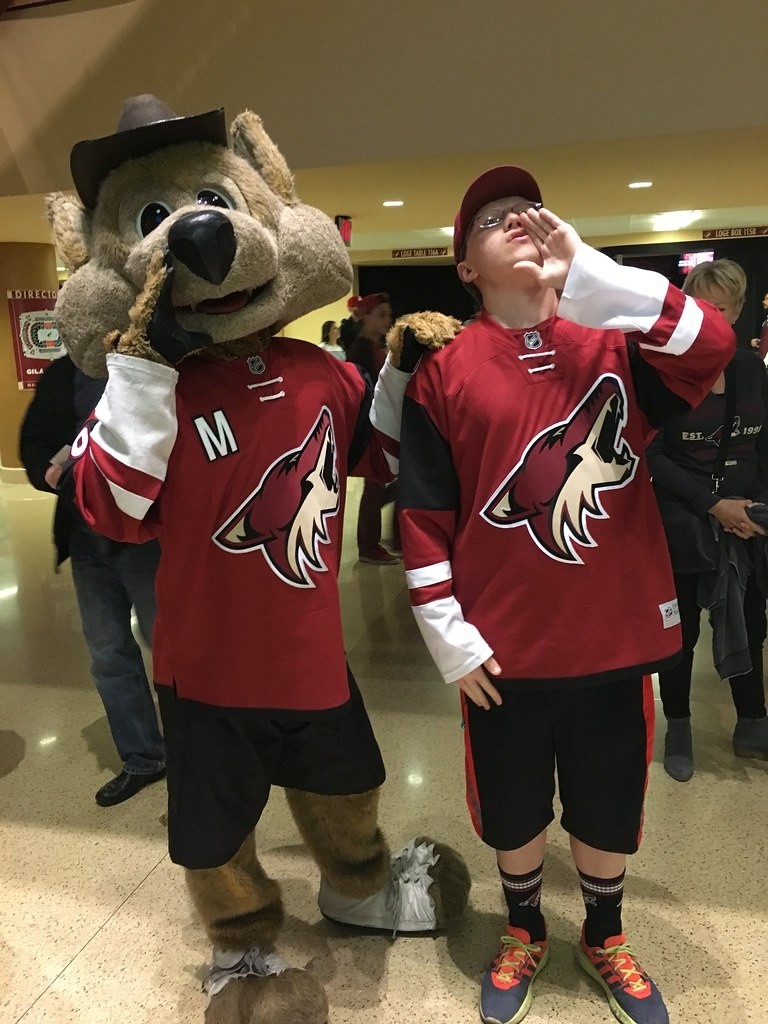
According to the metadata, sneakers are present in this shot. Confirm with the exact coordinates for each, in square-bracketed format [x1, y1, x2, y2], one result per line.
[575, 918, 669, 1024]
[479, 914, 550, 1024]
[358, 545, 400, 565]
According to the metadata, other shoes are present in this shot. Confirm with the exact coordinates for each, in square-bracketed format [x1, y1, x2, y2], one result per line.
[733, 716, 768, 761]
[664, 717, 694, 783]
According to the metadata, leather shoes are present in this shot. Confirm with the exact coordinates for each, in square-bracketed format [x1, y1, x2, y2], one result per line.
[95, 768, 166, 807]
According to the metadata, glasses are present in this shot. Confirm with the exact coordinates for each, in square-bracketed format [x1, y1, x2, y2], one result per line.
[462, 201, 541, 260]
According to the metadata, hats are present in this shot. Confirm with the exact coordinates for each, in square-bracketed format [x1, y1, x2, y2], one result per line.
[454, 166, 544, 265]
[348, 293, 390, 314]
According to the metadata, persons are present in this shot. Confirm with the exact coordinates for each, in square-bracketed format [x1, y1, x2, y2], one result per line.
[322, 292, 402, 567]
[648, 259, 768, 783]
[397, 165, 738, 1023]
[15, 356, 167, 807]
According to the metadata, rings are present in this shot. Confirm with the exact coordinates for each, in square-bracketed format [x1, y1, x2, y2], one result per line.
[740, 522, 750, 529]
[723, 527, 737, 532]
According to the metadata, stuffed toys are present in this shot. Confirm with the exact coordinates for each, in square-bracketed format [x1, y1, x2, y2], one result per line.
[46, 92, 470, 1024]
